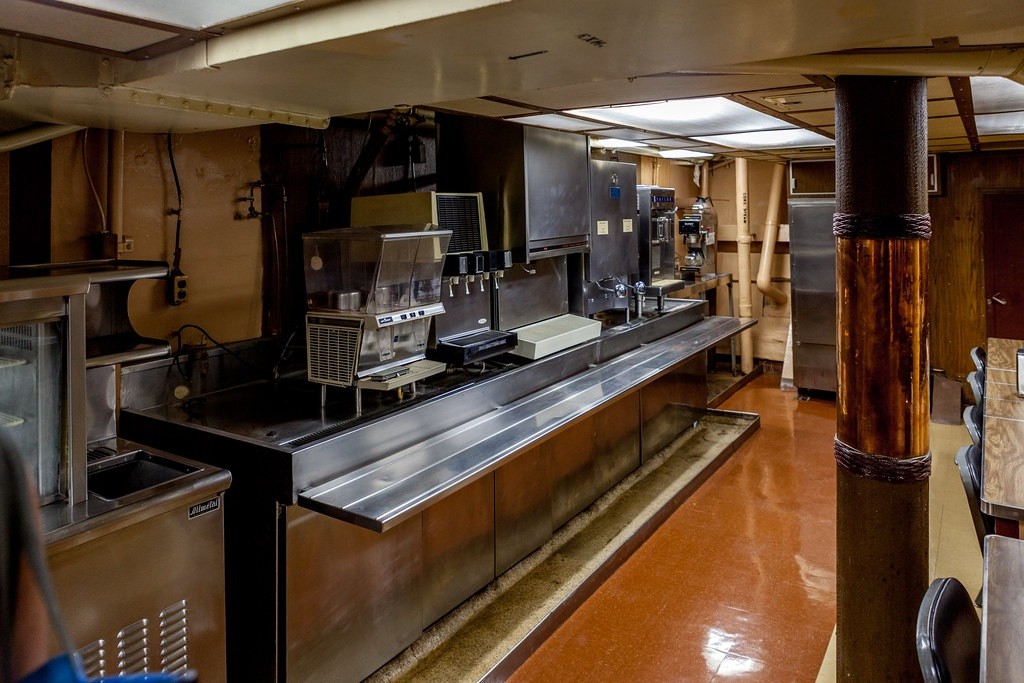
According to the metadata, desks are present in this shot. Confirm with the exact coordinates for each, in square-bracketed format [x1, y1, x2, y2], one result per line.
[979, 534, 1024, 683]
[981, 337, 1024, 523]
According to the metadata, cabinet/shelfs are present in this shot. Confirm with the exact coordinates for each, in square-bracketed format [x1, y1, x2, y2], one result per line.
[277, 350, 707, 683]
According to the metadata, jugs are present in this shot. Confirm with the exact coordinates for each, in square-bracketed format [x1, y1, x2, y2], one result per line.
[684, 247, 705, 266]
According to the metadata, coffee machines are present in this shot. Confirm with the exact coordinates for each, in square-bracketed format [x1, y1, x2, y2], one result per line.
[680, 197, 716, 280]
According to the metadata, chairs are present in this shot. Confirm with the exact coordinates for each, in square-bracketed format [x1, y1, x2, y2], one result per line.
[917, 345, 986, 683]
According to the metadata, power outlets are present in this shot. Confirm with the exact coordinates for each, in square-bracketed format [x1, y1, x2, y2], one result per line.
[167, 269, 188, 306]
